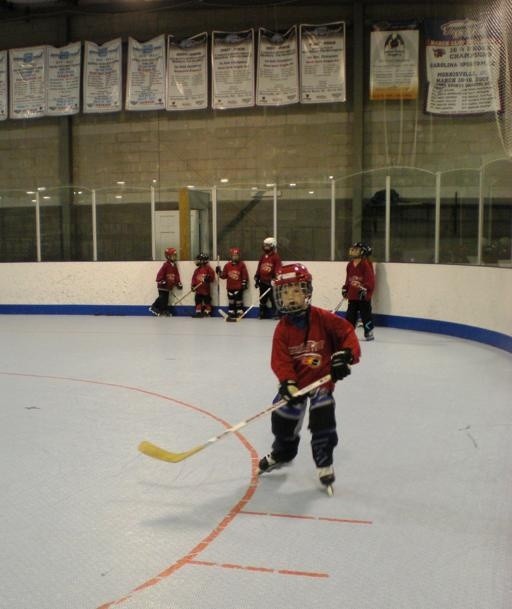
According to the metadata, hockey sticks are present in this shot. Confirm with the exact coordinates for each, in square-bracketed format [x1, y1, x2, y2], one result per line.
[218, 256, 229, 317]
[138, 372, 333, 463]
[226, 287, 271, 322]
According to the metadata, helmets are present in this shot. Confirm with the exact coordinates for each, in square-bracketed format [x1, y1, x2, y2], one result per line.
[230, 248, 240, 255]
[349, 241, 373, 259]
[193, 252, 209, 266]
[263, 237, 277, 248]
[165, 247, 176, 258]
[272, 263, 313, 314]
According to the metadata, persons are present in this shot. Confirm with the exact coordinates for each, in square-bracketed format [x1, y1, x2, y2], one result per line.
[259, 263, 362, 487]
[342, 242, 375, 341]
[148, 247, 182, 316]
[253, 237, 283, 319]
[190, 253, 215, 317]
[216, 246, 249, 322]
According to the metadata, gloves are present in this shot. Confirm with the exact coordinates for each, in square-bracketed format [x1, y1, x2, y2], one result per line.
[160, 280, 167, 287]
[342, 286, 346, 296]
[255, 280, 261, 288]
[329, 346, 352, 383]
[204, 276, 211, 282]
[358, 290, 366, 300]
[175, 282, 183, 289]
[279, 379, 307, 405]
[239, 281, 248, 290]
[191, 286, 196, 292]
[259, 454, 278, 470]
[215, 266, 222, 274]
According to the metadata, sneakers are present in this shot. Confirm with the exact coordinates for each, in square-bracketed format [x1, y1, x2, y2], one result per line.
[364, 331, 374, 341]
[316, 463, 335, 485]
[149, 304, 211, 316]
[227, 303, 275, 317]
